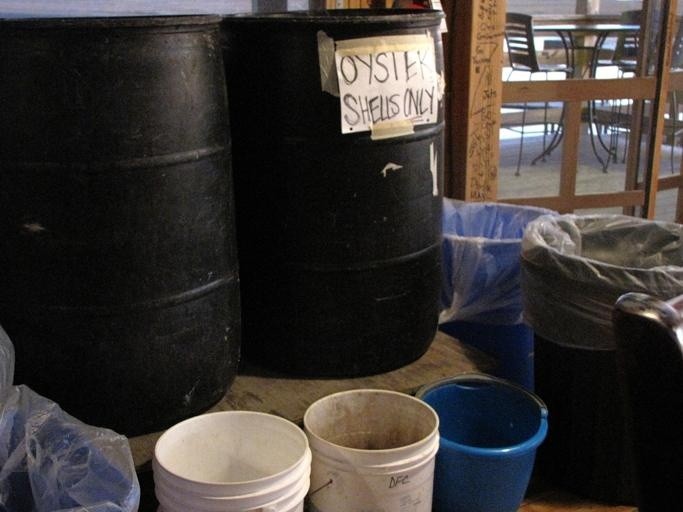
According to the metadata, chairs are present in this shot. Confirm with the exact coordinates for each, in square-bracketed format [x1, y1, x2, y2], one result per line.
[501, 12, 683, 177]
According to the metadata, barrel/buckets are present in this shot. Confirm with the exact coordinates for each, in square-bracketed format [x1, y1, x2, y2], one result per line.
[152, 411, 312, 511]
[0, 17, 244, 435]
[303, 388, 440, 512]
[220, 4, 446, 378]
[414, 369, 548, 512]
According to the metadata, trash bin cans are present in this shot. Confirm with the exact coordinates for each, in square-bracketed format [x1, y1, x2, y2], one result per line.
[610, 290, 683, 512]
[520, 213, 682, 508]
[0, 408, 136, 512]
[442, 196, 560, 394]
